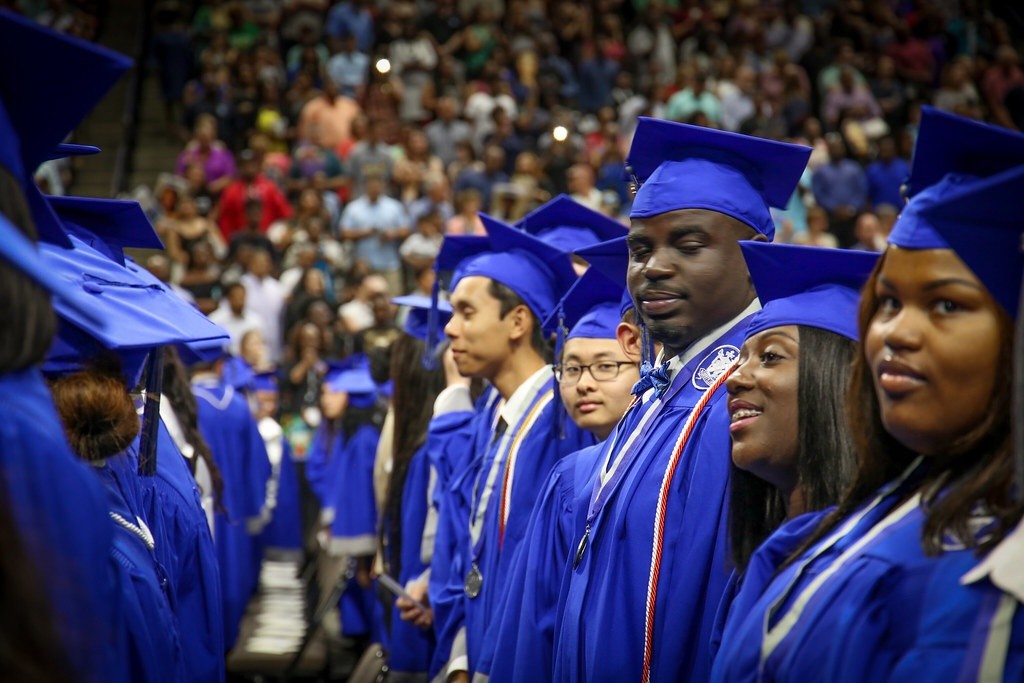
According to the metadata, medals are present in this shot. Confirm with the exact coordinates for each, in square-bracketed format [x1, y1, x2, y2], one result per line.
[465, 564, 483, 599]
[573, 533, 589, 570]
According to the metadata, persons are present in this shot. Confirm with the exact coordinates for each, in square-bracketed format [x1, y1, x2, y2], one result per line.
[0, 0, 1024, 683]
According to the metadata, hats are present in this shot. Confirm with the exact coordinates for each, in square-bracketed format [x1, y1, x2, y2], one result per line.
[541, 265, 624, 368]
[1, 12, 259, 391]
[463, 212, 577, 340]
[511, 192, 629, 256]
[738, 240, 884, 343]
[321, 356, 381, 408]
[572, 234, 655, 369]
[422, 235, 489, 369]
[918, 168, 1023, 313]
[627, 116, 814, 240]
[390, 296, 453, 343]
[886, 103, 1024, 251]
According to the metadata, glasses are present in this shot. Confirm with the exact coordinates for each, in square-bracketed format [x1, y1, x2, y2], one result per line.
[552, 359, 640, 382]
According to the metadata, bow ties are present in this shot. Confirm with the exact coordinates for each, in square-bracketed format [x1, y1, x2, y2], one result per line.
[630, 360, 672, 399]
